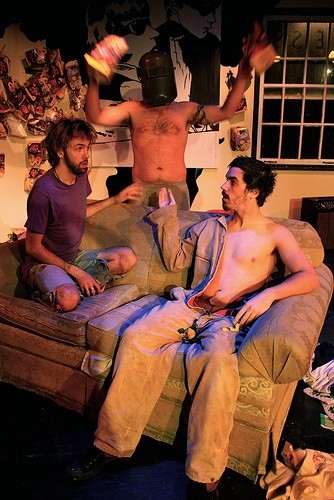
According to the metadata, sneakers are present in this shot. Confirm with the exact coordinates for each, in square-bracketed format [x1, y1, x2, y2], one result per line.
[66, 440, 123, 480]
[187, 477, 221, 500]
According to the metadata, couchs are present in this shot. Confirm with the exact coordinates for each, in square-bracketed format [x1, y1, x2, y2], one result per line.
[0, 199, 334, 486]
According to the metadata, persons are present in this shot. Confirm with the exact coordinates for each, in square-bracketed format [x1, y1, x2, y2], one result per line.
[64, 155, 320, 500]
[20, 117, 137, 313]
[83, 29, 265, 212]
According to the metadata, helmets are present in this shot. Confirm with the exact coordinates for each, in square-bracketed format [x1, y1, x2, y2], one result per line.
[136, 51, 178, 106]
